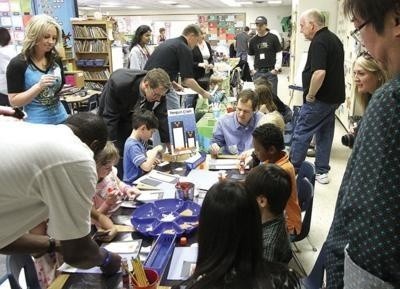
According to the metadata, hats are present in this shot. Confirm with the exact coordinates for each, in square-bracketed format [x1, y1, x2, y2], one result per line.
[256, 16, 267, 24]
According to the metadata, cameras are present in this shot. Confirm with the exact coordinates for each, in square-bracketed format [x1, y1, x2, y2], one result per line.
[342, 133, 354, 147]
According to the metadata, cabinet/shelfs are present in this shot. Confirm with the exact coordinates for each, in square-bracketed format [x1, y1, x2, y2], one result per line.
[70, 20, 114, 85]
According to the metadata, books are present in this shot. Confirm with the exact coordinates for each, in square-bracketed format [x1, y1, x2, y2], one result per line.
[72, 24, 108, 88]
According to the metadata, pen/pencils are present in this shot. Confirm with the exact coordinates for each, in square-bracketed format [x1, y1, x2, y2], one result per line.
[121, 255, 151, 287]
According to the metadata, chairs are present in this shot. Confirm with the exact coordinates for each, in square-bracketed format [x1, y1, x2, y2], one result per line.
[280, 160, 319, 252]
[73, 93, 99, 113]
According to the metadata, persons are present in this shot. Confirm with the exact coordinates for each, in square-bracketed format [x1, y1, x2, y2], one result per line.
[91, 140, 142, 243]
[6, 13, 72, 125]
[0, 112, 122, 276]
[287, 6, 347, 186]
[322, 1, 400, 289]
[347, 50, 386, 140]
[0, 104, 26, 120]
[237, 123, 302, 242]
[99, 11, 294, 181]
[243, 162, 295, 266]
[0, 27, 19, 107]
[170, 180, 315, 289]
[27, 211, 118, 289]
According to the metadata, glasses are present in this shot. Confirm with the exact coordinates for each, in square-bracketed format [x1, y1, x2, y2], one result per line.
[350, 18, 370, 42]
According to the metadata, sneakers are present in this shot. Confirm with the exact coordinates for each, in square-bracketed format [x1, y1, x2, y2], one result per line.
[315, 173, 329, 183]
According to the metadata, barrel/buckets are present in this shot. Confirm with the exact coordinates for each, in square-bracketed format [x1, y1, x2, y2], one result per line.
[175, 181, 196, 200]
[129, 266, 160, 289]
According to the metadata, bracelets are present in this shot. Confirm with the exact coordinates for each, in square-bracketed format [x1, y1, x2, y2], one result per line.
[100, 250, 113, 268]
[49, 236, 58, 253]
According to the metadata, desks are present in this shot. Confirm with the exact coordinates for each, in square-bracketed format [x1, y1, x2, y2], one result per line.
[195, 112, 217, 139]
[49, 154, 234, 289]
[64, 90, 103, 113]
